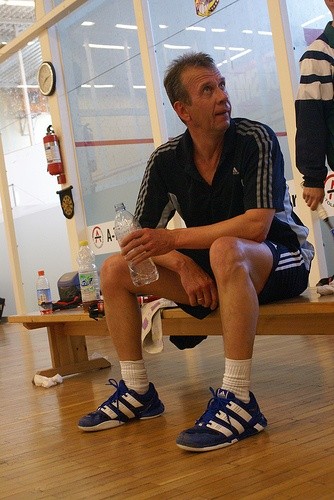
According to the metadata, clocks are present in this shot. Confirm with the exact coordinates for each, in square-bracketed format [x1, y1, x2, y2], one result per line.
[38, 62, 55, 96]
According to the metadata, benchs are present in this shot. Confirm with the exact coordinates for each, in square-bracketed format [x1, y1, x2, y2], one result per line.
[9, 287, 334, 386]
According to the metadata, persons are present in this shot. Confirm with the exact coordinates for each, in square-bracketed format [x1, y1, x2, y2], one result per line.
[294, 0, 334, 210]
[76, 52, 315, 453]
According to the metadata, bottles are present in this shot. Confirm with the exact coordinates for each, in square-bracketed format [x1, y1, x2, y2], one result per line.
[77, 240, 100, 302]
[113, 203, 160, 286]
[36, 270, 52, 310]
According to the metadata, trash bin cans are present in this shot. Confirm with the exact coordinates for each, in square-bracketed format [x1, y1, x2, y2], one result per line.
[56, 271, 82, 304]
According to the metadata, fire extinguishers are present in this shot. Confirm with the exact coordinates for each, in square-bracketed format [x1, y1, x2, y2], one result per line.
[42, 125, 64, 175]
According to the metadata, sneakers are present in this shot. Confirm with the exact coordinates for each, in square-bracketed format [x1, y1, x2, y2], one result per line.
[78, 378, 165, 432]
[175, 385, 268, 452]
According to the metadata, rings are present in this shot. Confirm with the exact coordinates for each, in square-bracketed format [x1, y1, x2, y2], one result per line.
[197, 297, 204, 301]
